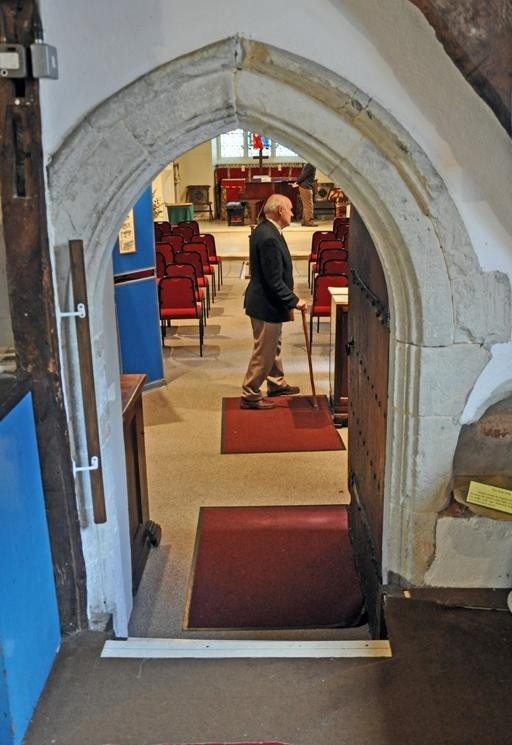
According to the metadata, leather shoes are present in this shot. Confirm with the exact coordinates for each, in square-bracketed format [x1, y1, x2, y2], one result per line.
[267, 384, 300, 397]
[239, 395, 276, 409]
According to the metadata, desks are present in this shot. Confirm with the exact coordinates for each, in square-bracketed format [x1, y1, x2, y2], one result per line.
[244, 177, 300, 226]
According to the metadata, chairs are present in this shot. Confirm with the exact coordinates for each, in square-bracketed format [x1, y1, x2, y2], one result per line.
[312, 182, 336, 219]
[307, 214, 349, 351]
[154, 220, 223, 360]
[184, 185, 214, 221]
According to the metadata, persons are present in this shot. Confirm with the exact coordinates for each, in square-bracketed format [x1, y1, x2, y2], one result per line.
[293, 162, 319, 227]
[237, 193, 309, 409]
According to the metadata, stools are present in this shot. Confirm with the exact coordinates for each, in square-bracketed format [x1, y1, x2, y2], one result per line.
[226, 200, 244, 226]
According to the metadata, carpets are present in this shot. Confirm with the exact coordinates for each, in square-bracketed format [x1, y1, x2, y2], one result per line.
[221, 393, 345, 454]
[180, 502, 367, 633]
